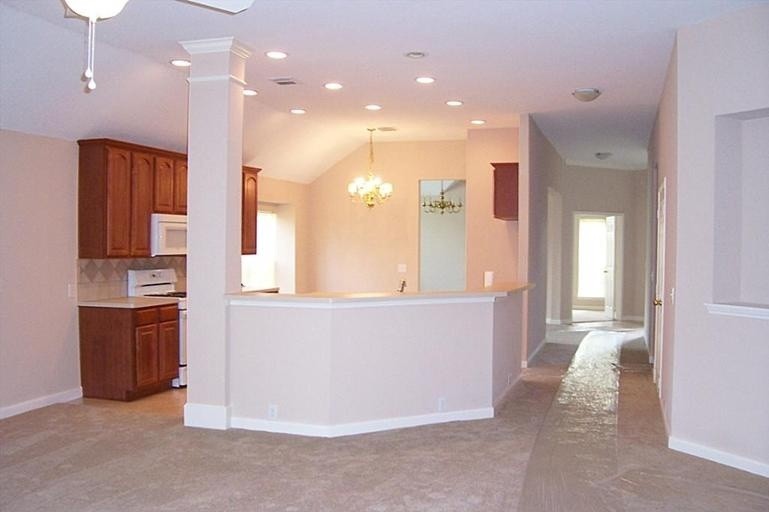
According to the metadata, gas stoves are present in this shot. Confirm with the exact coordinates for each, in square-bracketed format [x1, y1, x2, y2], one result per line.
[127, 290, 188, 309]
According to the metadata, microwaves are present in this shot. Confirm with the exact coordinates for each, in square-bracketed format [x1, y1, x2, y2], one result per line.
[151, 214, 187, 258]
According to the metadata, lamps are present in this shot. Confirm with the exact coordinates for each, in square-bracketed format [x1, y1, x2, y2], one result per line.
[63, 0, 130, 20]
[348, 129, 394, 209]
[422, 180, 463, 215]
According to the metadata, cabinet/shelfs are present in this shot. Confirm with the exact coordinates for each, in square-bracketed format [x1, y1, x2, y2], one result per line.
[78, 139, 258, 260]
[79, 305, 179, 402]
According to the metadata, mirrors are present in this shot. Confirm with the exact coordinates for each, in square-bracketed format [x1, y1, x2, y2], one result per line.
[420, 180, 466, 293]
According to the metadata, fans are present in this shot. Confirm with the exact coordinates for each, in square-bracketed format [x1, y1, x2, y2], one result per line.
[58, 0, 253, 14]
